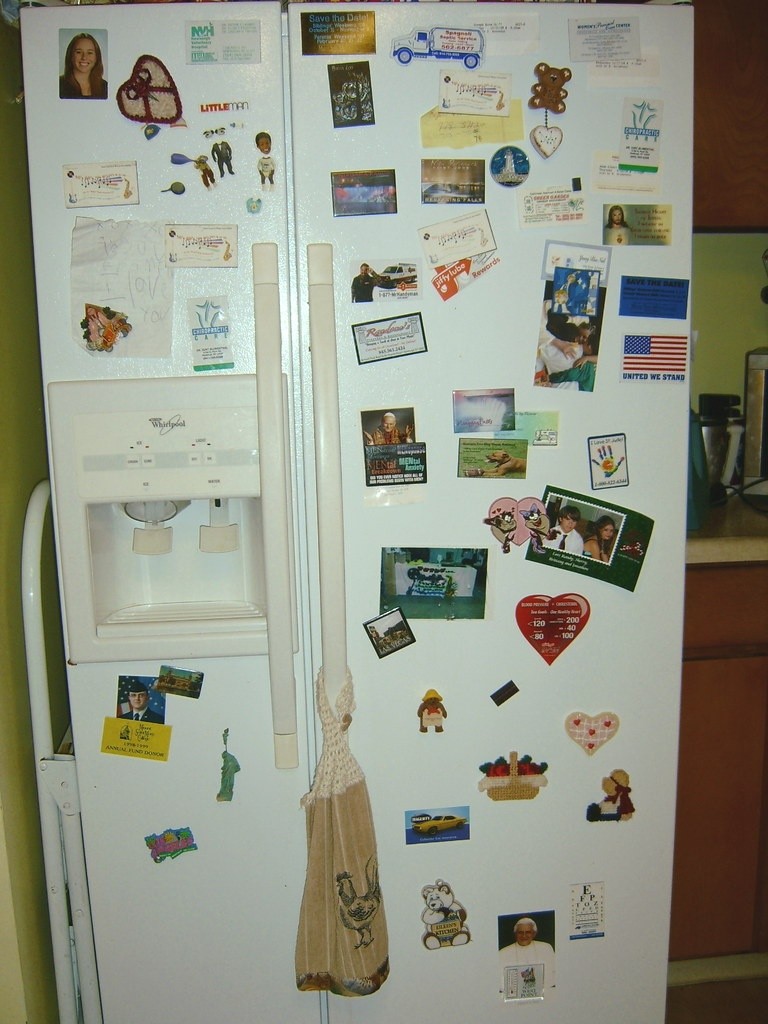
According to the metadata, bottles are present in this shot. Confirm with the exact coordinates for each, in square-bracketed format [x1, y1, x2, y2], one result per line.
[687, 395, 709, 531]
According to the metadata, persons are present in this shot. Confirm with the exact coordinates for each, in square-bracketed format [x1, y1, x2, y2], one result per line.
[117, 680, 165, 724]
[59, 28, 108, 100]
[540, 506, 585, 556]
[362, 412, 414, 446]
[255, 133, 271, 155]
[603, 205, 634, 245]
[498, 917, 555, 984]
[538, 300, 599, 391]
[351, 263, 391, 303]
[583, 514, 615, 562]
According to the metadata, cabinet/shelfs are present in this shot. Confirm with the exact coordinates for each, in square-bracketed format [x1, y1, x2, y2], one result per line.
[666, 561, 768, 987]
[692, 0, 768, 231]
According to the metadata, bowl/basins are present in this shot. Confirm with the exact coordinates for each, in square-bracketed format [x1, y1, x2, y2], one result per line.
[462, 468, 485, 477]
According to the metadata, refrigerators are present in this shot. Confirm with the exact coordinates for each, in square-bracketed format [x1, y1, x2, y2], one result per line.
[19, 1, 697, 1024]
